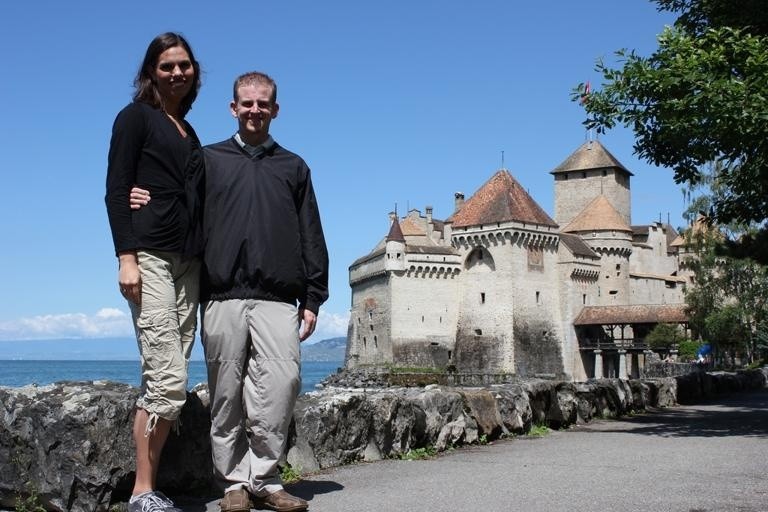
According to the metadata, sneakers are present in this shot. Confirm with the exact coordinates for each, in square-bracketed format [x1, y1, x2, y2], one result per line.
[253, 491, 308, 512]
[128, 490, 183, 512]
[222, 488, 252, 512]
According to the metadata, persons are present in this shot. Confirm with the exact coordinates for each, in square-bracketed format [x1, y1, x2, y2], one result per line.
[129, 73, 330, 512]
[103, 33, 206, 511]
